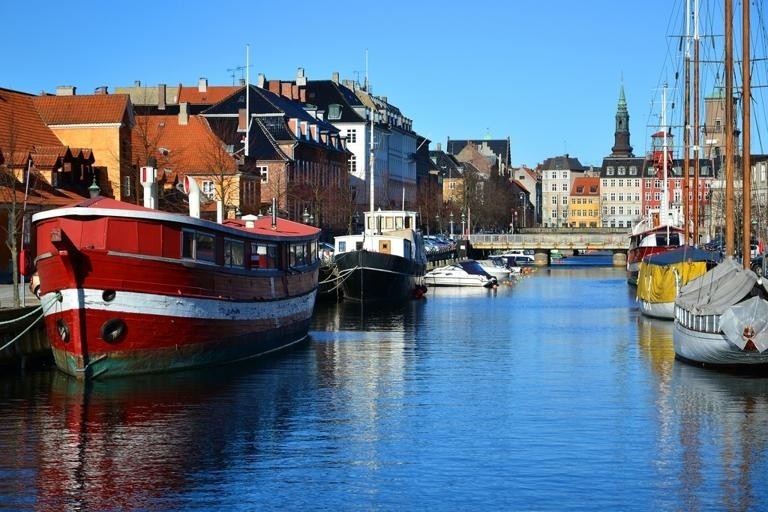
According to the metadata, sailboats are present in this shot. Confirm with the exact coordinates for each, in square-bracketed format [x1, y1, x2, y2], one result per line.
[625, 80, 703, 286]
[672, 1, 768, 366]
[636, 2, 727, 320]
[704, 7, 768, 297]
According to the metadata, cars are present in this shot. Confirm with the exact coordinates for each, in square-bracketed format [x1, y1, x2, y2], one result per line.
[423, 233, 456, 252]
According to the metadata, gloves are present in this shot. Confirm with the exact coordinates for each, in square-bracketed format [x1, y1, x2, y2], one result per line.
[333, 209, 426, 303]
[424, 250, 535, 286]
[32, 165, 322, 378]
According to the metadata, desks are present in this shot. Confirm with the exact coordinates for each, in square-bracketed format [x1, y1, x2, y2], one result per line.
[511, 208, 517, 234]
[461, 212, 467, 234]
[449, 212, 455, 234]
[520, 192, 526, 227]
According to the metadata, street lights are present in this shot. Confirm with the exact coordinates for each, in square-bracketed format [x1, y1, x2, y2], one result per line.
[511, 208, 517, 234]
[449, 212, 455, 234]
[461, 212, 467, 234]
[520, 192, 526, 227]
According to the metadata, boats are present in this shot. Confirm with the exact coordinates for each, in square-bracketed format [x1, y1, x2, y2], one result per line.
[32, 165, 322, 378]
[424, 250, 535, 286]
[333, 209, 426, 303]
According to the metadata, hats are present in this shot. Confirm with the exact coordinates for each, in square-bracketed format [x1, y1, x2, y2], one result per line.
[423, 233, 456, 252]
